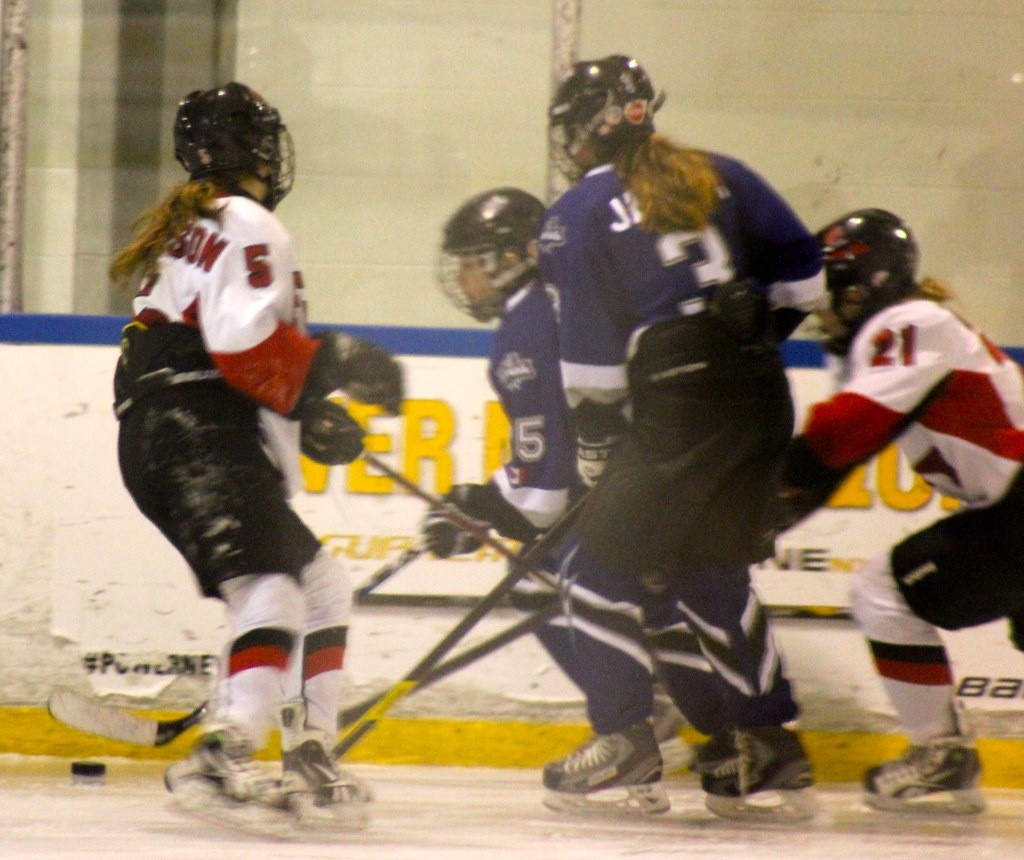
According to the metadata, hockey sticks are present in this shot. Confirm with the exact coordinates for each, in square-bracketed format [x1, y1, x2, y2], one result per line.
[336, 601, 565, 730]
[327, 485, 601, 766]
[357, 450, 562, 593]
[44, 530, 440, 750]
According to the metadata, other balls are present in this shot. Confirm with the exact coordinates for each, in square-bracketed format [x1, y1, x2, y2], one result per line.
[70, 760, 107, 778]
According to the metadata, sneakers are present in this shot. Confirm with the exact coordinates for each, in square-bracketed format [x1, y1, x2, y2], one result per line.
[541, 715, 671, 816]
[165, 723, 308, 841]
[696, 721, 818, 826]
[864, 696, 985, 823]
[646, 686, 692, 778]
[280, 705, 372, 820]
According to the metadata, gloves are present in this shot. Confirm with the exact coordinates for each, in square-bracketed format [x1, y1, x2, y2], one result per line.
[312, 329, 399, 415]
[425, 484, 491, 557]
[773, 436, 852, 531]
[300, 400, 366, 465]
[570, 404, 632, 489]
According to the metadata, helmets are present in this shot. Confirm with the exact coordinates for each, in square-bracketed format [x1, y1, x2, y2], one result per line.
[817, 209, 918, 325]
[174, 83, 295, 210]
[437, 188, 549, 322]
[547, 55, 656, 185]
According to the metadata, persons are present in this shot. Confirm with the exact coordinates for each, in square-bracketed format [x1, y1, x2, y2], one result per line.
[425, 54, 1023, 824]
[108, 81, 401, 836]
